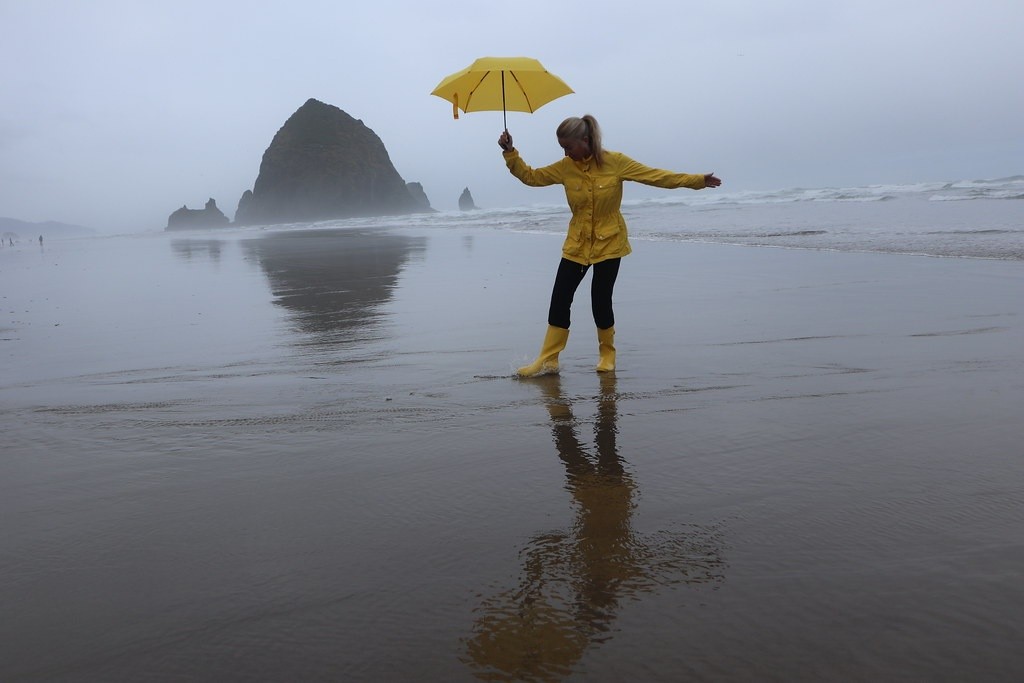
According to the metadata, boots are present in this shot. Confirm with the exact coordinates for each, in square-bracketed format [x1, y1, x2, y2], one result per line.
[596, 324, 616, 372]
[516, 324, 570, 376]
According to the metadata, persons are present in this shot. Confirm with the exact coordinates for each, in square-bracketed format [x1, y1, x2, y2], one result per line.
[498, 114, 723, 379]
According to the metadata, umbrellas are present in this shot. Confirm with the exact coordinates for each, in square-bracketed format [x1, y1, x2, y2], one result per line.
[430, 55, 575, 144]
